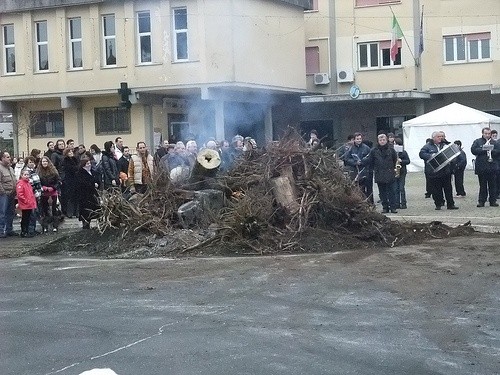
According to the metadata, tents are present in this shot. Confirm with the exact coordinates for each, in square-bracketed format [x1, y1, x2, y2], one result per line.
[402, 101, 500, 173]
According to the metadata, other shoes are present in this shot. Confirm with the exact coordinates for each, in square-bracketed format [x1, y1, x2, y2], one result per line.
[44, 228, 47, 231]
[5, 230, 19, 236]
[425, 194, 430, 198]
[456, 192, 466, 196]
[53, 228, 57, 232]
[400, 205, 406, 209]
[447, 205, 459, 209]
[391, 209, 397, 213]
[477, 201, 484, 207]
[382, 209, 389, 213]
[0, 233, 6, 237]
[435, 206, 441, 210]
[68, 215, 72, 218]
[490, 201, 499, 206]
[76, 215, 79, 217]
[21, 231, 40, 238]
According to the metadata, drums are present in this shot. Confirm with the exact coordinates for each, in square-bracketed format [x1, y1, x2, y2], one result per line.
[426, 142, 462, 173]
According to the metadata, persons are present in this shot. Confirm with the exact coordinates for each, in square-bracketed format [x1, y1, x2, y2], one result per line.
[101, 140, 122, 193]
[471, 127, 499, 207]
[340, 132, 373, 204]
[36, 156, 63, 234]
[0, 135, 259, 237]
[361, 130, 398, 213]
[15, 168, 38, 237]
[127, 141, 156, 196]
[0, 150, 20, 237]
[75, 159, 103, 230]
[419, 131, 459, 210]
[305, 130, 499, 208]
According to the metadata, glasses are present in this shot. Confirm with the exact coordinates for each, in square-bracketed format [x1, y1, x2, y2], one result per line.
[138, 147, 145, 149]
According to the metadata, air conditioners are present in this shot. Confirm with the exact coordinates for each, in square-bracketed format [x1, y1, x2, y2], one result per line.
[313, 73, 330, 85]
[337, 68, 354, 83]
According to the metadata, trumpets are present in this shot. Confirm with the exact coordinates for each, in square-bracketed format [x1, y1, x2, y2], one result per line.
[395, 152, 402, 178]
[485, 139, 493, 163]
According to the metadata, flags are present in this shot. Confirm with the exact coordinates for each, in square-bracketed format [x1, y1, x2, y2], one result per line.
[391, 16, 424, 63]
